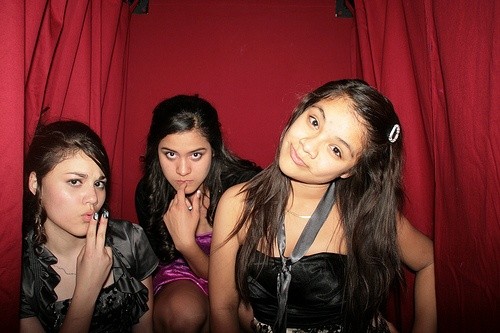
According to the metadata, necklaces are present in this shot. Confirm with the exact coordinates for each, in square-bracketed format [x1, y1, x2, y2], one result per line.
[53, 264, 77, 277]
[286, 209, 311, 218]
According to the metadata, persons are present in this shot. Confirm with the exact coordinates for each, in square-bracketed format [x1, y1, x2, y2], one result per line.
[209, 78, 437, 333]
[19, 120, 158, 333]
[135, 94, 264, 333]
